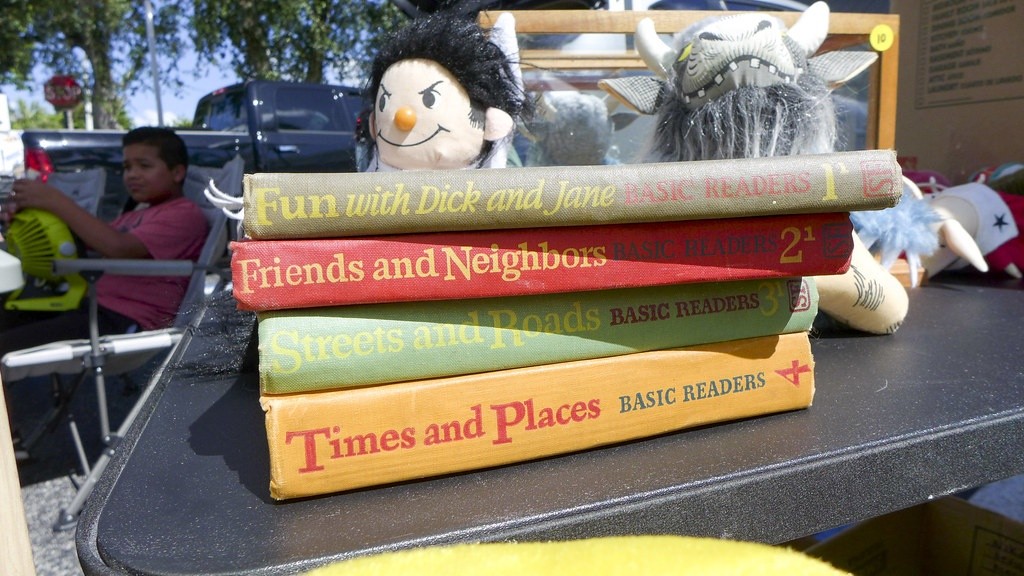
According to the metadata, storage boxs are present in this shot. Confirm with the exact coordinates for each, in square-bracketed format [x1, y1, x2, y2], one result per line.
[888, 0, 1024, 186]
[777, 494, 1024, 576]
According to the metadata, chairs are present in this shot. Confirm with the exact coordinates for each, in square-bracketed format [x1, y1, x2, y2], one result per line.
[0, 151, 244, 490]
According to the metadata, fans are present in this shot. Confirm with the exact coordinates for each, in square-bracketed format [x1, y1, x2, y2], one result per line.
[4, 207, 88, 311]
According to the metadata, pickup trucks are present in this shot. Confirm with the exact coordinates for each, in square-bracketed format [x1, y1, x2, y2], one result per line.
[21, 77, 375, 232]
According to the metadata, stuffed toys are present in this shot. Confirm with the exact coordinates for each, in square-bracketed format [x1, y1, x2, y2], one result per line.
[354, 0, 1024, 335]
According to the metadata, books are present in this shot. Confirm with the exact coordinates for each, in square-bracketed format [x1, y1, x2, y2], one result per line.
[228, 147, 905, 502]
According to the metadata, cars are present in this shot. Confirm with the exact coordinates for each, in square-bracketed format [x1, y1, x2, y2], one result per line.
[505, 0, 869, 168]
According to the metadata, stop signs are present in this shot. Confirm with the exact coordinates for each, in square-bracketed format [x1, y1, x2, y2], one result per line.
[44, 74, 84, 111]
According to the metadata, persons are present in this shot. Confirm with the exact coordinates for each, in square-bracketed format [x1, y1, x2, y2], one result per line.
[0, 126, 210, 461]
